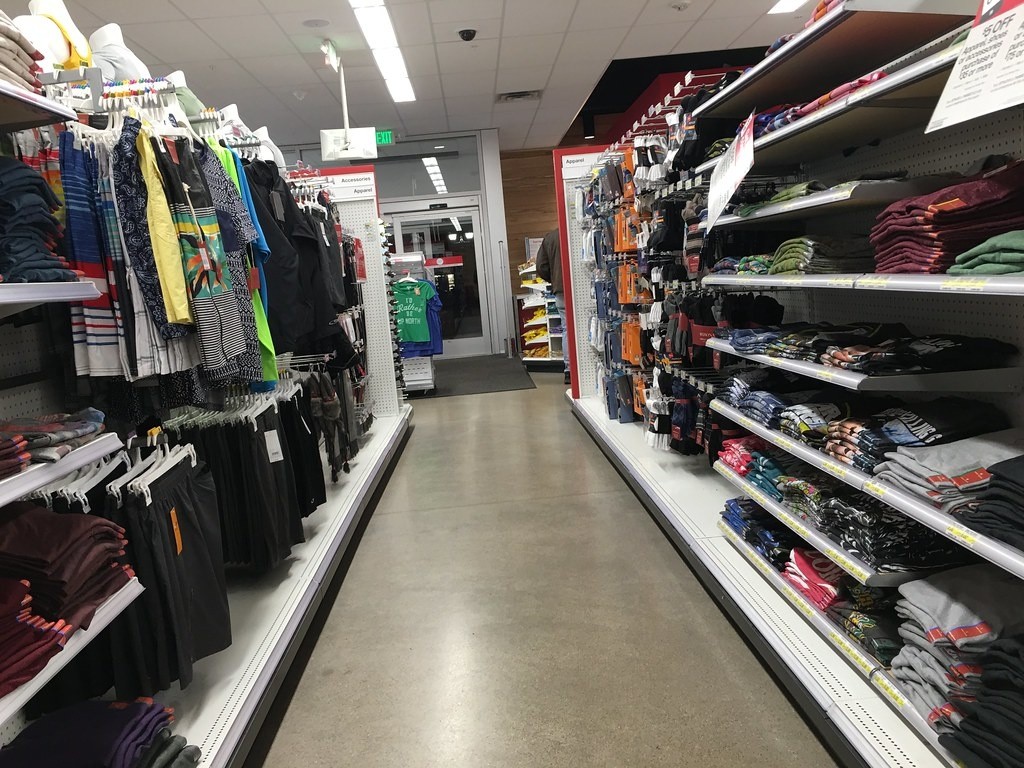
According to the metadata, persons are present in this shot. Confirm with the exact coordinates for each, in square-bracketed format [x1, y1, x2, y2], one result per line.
[536, 228, 571, 385]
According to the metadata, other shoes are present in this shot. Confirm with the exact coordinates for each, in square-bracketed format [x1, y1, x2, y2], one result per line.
[565, 371, 571, 384]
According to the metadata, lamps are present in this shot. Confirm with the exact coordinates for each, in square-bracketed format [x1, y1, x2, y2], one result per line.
[583, 111, 596, 140]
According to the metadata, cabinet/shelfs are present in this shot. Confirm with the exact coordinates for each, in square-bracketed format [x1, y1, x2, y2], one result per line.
[516, 260, 564, 374]
[704, 2, 1024, 768]
[1, 80, 149, 724]
